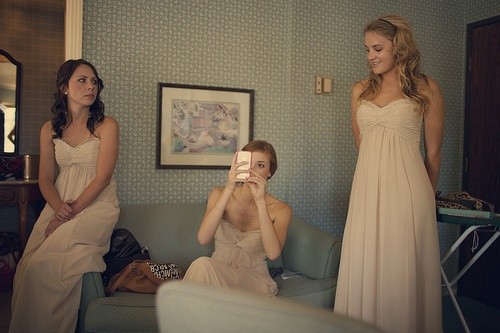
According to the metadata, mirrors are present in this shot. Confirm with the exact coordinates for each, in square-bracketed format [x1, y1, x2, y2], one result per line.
[0, 49, 21, 156]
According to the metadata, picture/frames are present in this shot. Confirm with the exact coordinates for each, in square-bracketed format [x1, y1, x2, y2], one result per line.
[156, 82, 255, 170]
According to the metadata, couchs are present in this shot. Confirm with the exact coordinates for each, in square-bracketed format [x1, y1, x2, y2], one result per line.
[79, 203, 342, 333]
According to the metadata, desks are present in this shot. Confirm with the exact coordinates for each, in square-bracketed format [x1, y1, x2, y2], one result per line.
[436, 213, 500, 333]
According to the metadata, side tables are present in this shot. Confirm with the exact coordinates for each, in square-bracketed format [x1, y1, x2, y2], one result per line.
[0, 180, 41, 258]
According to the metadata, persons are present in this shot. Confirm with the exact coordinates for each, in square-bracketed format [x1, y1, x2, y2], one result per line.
[8, 128, 14, 144]
[334, 15, 444, 333]
[8, 59, 120, 333]
[182, 141, 292, 297]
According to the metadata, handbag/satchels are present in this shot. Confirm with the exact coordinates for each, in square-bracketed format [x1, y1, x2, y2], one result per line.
[105, 260, 181, 296]
[100, 229, 149, 284]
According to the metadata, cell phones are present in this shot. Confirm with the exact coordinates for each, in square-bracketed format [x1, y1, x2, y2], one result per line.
[236, 151, 252, 179]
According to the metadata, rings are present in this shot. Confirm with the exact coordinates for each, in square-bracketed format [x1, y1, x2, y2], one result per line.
[59, 212, 62, 215]
